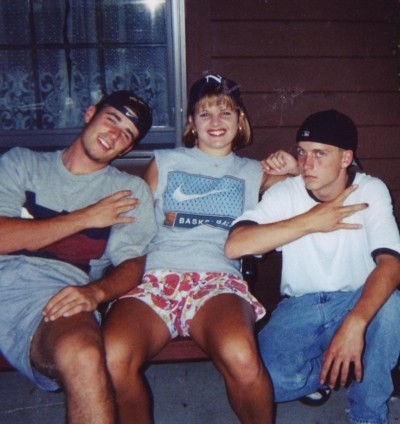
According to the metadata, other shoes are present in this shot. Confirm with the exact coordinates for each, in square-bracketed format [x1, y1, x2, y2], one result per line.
[299, 388, 333, 406]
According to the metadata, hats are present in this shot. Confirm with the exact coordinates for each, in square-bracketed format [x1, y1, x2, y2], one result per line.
[297, 111, 366, 173]
[103, 90, 153, 137]
[189, 75, 240, 103]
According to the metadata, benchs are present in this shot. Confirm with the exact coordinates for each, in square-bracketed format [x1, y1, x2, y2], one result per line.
[0, 249, 289, 370]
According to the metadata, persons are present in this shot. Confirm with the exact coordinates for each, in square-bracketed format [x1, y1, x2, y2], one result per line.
[100, 74, 300, 424]
[0, 86, 157, 423]
[225, 110, 400, 424]
